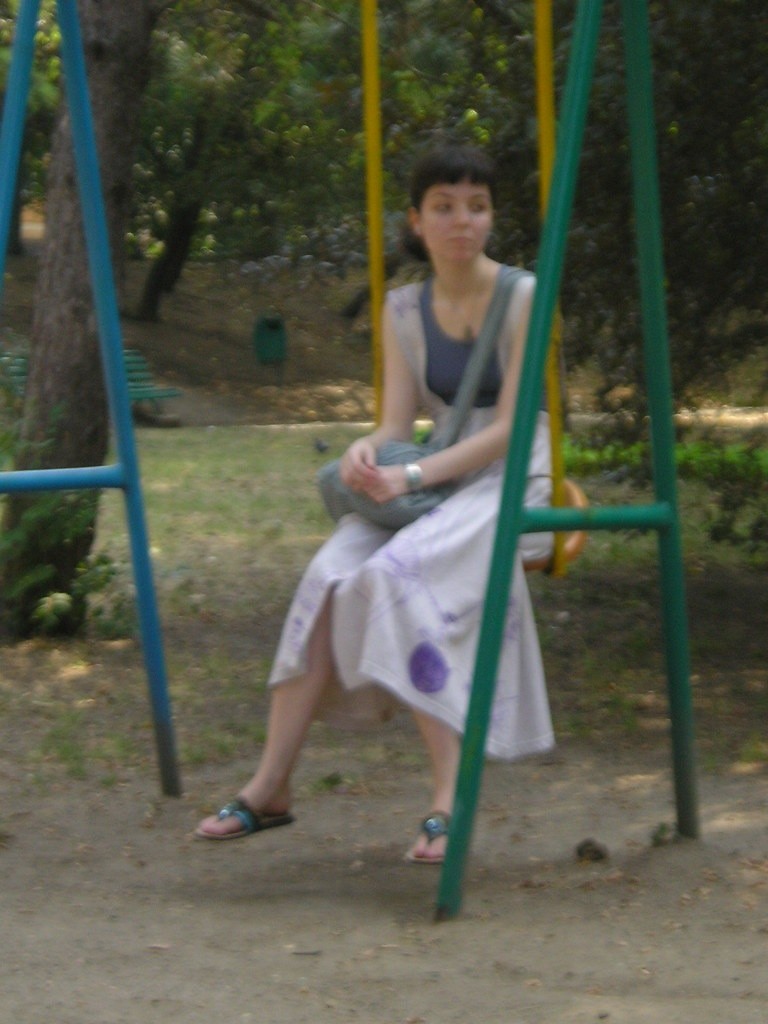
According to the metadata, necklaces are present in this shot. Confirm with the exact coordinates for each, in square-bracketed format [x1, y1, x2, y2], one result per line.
[433, 278, 485, 344]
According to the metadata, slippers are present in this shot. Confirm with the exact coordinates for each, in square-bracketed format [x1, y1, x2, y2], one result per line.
[193, 796, 296, 840]
[404, 809, 450, 862]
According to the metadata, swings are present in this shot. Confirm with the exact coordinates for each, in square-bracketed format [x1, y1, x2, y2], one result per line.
[362, 1, 590, 577]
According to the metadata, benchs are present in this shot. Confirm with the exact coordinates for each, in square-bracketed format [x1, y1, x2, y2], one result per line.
[0, 347, 182, 415]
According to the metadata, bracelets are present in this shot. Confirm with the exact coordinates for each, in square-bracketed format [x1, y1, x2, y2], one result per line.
[406, 463, 424, 491]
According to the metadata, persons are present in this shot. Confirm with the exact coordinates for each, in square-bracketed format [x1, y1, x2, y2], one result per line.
[196, 149, 554, 863]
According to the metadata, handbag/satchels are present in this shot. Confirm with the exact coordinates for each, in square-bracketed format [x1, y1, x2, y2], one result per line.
[315, 440, 454, 531]
